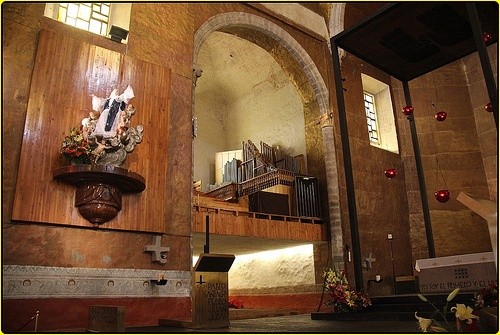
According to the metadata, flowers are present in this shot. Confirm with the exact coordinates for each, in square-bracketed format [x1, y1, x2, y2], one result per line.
[413, 281, 498, 334]
[322, 264, 372, 312]
[56, 128, 95, 166]
